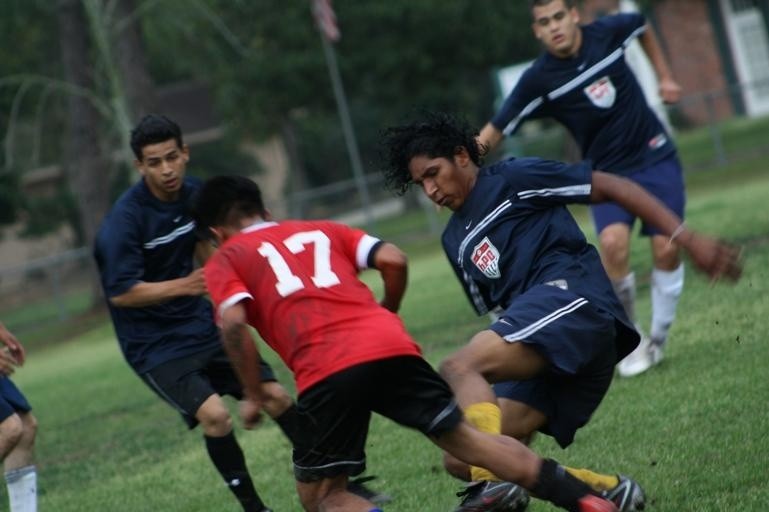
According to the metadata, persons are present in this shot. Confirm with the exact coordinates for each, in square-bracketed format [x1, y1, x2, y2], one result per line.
[0, 318, 40, 512]
[469, 1, 687, 381]
[374, 111, 744, 512]
[197, 171, 621, 512]
[93, 112, 393, 511]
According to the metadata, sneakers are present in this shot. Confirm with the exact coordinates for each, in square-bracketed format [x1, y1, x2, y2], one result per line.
[453, 477, 531, 512]
[577, 472, 646, 512]
[618, 342, 665, 377]
